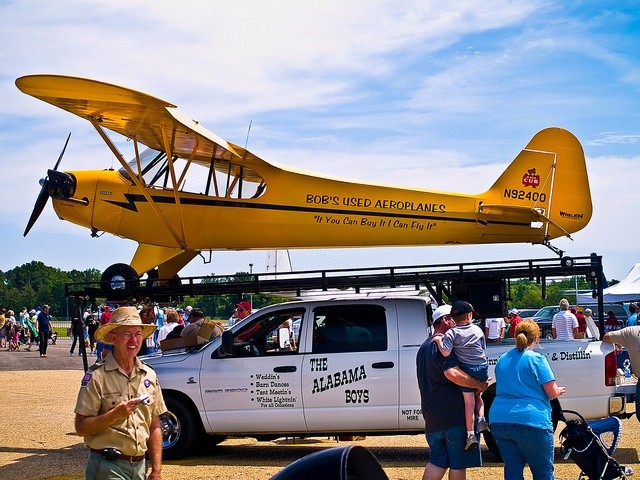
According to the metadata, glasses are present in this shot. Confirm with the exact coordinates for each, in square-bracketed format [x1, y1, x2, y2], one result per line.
[237, 308, 245, 312]
[115, 331, 145, 340]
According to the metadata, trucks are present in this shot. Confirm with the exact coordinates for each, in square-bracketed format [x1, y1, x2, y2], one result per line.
[523, 304, 628, 340]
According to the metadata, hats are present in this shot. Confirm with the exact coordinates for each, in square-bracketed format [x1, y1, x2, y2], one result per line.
[560, 298, 569, 310]
[43, 304, 51, 308]
[168, 310, 180, 322]
[510, 309, 518, 314]
[94, 306, 158, 345]
[29, 309, 36, 314]
[177, 309, 185, 313]
[452, 301, 480, 316]
[187, 308, 203, 322]
[432, 305, 452, 323]
[231, 301, 251, 314]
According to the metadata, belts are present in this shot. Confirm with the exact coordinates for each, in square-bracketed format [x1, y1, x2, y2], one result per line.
[89, 447, 146, 464]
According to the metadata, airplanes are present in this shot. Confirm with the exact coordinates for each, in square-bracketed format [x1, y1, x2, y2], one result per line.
[15, 75, 593, 299]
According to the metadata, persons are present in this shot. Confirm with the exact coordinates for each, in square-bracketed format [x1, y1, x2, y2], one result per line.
[181, 308, 222, 338]
[99, 306, 112, 325]
[433, 301, 491, 451]
[20, 306, 30, 329]
[504, 317, 511, 339]
[229, 309, 238, 327]
[603, 324, 640, 422]
[236, 301, 262, 342]
[36, 305, 52, 358]
[485, 318, 505, 343]
[628, 304, 638, 326]
[570, 308, 586, 339]
[585, 308, 592, 316]
[488, 318, 566, 479]
[509, 308, 523, 338]
[416, 304, 492, 480]
[0, 304, 21, 351]
[178, 309, 185, 327]
[24, 308, 42, 351]
[69, 309, 85, 357]
[36, 306, 42, 315]
[551, 299, 579, 340]
[135, 308, 155, 356]
[604, 311, 619, 325]
[86, 309, 100, 357]
[157, 309, 180, 347]
[74, 306, 168, 480]
[278, 317, 297, 351]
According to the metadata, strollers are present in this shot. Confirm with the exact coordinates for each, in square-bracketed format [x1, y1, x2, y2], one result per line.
[558, 410, 634, 480]
[267, 444, 391, 480]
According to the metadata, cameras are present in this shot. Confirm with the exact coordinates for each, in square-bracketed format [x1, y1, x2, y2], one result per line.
[133, 393, 150, 406]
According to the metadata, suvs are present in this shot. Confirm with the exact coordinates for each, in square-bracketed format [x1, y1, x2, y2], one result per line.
[513, 309, 538, 318]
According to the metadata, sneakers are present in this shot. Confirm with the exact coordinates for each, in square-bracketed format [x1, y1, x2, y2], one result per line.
[465, 434, 478, 451]
[477, 419, 490, 433]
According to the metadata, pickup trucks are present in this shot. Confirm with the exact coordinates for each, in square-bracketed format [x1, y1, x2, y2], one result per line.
[137, 296, 627, 460]
